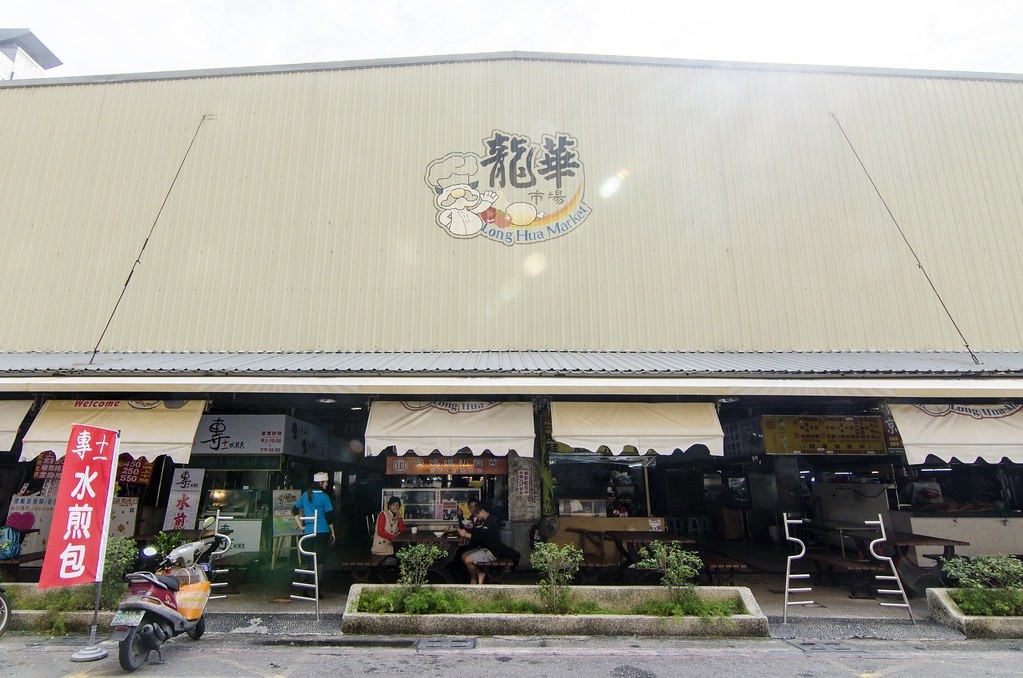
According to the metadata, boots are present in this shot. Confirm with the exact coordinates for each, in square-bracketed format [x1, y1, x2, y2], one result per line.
[299, 563, 310, 595]
[313, 564, 323, 600]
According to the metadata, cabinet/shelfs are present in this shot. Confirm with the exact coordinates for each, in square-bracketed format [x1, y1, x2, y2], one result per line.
[381, 490, 481, 521]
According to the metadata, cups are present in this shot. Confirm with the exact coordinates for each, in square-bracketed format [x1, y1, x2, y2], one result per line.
[411, 526, 417, 534]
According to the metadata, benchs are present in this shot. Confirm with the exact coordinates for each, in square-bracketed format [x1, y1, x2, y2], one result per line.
[213, 551, 265, 595]
[473, 542, 520, 584]
[695, 556, 746, 585]
[340, 549, 388, 582]
[809, 554, 884, 600]
[0, 551, 47, 582]
[580, 551, 606, 584]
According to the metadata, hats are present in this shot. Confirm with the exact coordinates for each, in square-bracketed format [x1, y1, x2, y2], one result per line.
[314, 472, 329, 481]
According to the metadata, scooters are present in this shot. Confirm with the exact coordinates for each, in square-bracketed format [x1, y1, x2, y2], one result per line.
[109, 516, 234, 672]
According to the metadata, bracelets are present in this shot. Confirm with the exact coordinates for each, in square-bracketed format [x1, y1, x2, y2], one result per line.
[464, 532, 467, 537]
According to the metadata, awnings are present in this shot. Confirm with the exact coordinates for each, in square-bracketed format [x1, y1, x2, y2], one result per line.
[364, 400, 536, 458]
[883, 402, 1023, 467]
[0, 400, 39, 452]
[16, 399, 210, 465]
[549, 401, 725, 457]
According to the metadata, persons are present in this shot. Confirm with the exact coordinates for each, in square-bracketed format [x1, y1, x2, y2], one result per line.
[458, 500, 502, 583]
[443, 498, 481, 583]
[371, 496, 410, 582]
[291, 471, 336, 600]
[604, 490, 628, 517]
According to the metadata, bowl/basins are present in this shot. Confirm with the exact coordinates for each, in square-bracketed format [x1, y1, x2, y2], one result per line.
[433, 531, 445, 537]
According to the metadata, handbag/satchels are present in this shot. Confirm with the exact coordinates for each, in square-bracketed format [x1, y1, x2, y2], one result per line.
[173, 568, 210, 621]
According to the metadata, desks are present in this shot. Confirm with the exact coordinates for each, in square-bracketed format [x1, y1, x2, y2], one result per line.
[792, 517, 881, 556]
[844, 530, 969, 609]
[607, 530, 696, 585]
[393, 529, 461, 583]
[134, 529, 217, 576]
